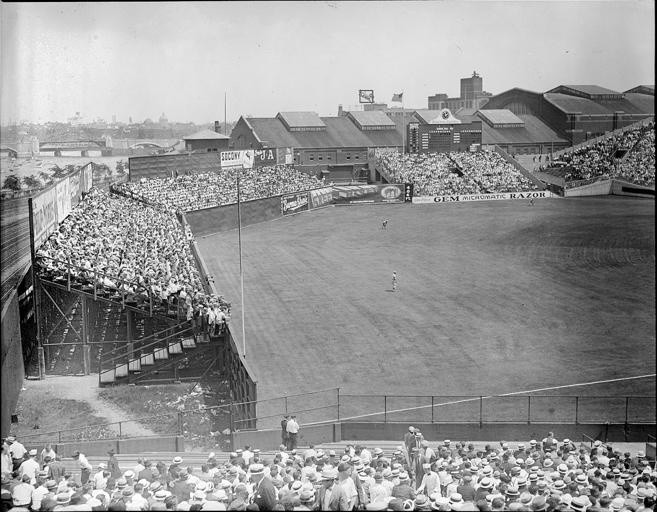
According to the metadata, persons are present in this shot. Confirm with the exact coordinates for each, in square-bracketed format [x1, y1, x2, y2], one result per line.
[286, 416, 300, 450]
[31, 163, 336, 336]
[391, 270, 398, 292]
[374, 117, 656, 198]
[0, 427, 656, 511]
[528, 198, 535, 207]
[280, 414, 290, 451]
[381, 219, 388, 230]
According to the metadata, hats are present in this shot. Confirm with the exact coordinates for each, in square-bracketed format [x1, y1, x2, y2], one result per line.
[408, 425, 415, 433]
[5, 435, 16, 443]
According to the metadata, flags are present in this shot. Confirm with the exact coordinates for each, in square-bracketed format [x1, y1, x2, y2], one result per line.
[391, 92, 402, 103]
